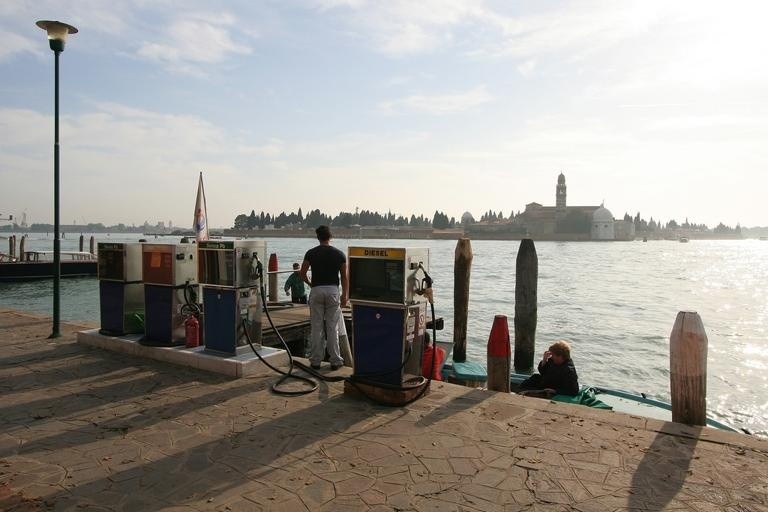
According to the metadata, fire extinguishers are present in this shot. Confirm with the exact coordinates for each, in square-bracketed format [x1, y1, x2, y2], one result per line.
[184, 312, 199, 348]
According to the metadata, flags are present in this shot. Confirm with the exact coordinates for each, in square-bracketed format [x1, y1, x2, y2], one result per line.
[193, 174, 207, 244]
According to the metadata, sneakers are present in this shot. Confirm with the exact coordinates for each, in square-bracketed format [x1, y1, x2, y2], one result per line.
[331, 364, 343, 370]
[311, 363, 320, 370]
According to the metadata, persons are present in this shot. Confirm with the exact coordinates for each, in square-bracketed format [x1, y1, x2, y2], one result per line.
[284, 263, 307, 304]
[420, 331, 445, 380]
[300, 225, 349, 370]
[516, 341, 580, 397]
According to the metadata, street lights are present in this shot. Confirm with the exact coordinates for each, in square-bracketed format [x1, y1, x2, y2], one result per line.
[36, 21, 79, 338]
